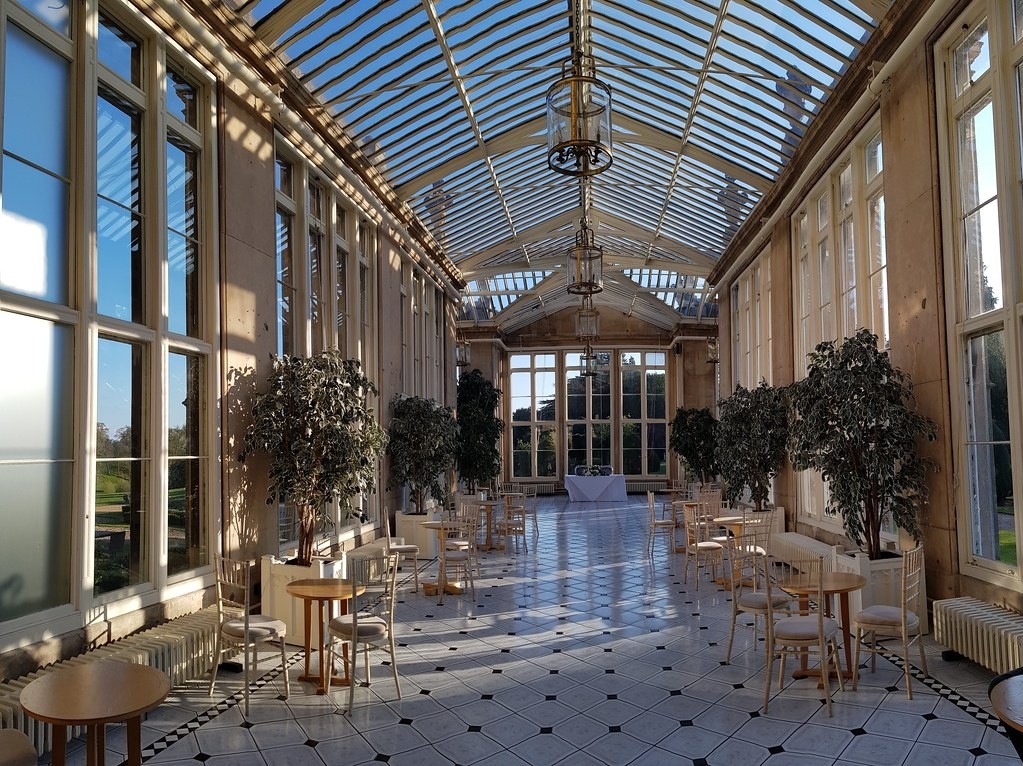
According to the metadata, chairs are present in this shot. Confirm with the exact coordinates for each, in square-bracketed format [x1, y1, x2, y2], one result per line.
[645, 478, 930, 720]
[0, 486, 543, 766]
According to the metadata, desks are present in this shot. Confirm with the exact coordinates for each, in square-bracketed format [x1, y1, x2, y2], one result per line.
[778, 571, 867, 690]
[711, 515, 761, 592]
[471, 499, 507, 551]
[563, 475, 627, 503]
[659, 487, 694, 534]
[285, 578, 365, 695]
[419, 521, 471, 604]
[19, 659, 170, 766]
[989, 674, 1023, 764]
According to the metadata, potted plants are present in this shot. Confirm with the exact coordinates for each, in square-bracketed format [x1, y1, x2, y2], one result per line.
[233, 347, 389, 653]
[453, 368, 510, 528]
[788, 323, 941, 647]
[668, 406, 724, 520]
[384, 393, 463, 561]
[713, 376, 797, 555]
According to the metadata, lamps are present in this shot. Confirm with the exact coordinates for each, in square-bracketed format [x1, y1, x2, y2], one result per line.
[574, 285, 600, 376]
[546, 0, 612, 177]
[567, 175, 605, 295]
